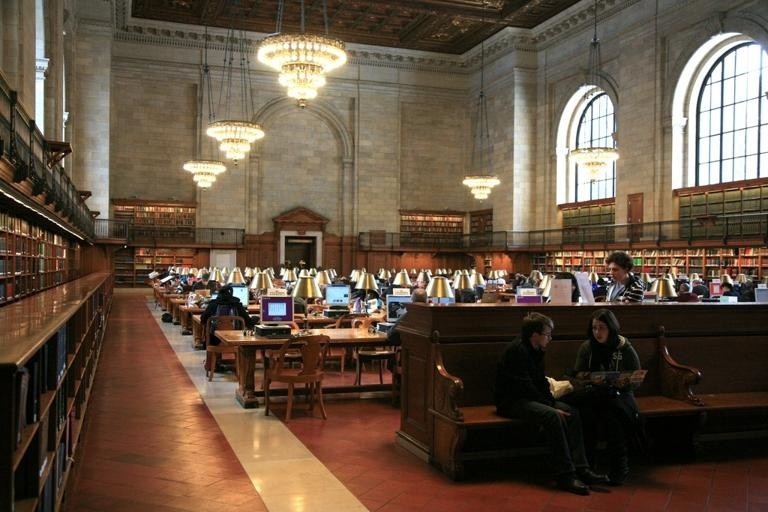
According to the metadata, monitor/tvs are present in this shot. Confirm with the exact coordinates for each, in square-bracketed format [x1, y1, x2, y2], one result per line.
[515, 295, 543, 303]
[432, 297, 449, 303]
[386, 295, 413, 322]
[231, 285, 249, 306]
[516, 286, 539, 295]
[260, 295, 294, 326]
[392, 287, 410, 296]
[754, 288, 768, 302]
[325, 285, 351, 308]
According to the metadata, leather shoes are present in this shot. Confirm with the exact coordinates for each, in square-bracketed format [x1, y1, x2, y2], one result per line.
[616, 456, 630, 483]
[559, 476, 589, 494]
[582, 468, 610, 485]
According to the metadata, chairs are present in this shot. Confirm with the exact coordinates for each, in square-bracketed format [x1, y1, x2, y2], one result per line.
[153, 276, 401, 424]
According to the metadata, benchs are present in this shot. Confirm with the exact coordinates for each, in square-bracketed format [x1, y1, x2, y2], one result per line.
[395, 301, 768, 481]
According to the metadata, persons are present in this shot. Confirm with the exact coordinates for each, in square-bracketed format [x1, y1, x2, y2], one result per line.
[543, 272, 581, 305]
[190, 273, 217, 291]
[719, 282, 741, 302]
[179, 273, 198, 293]
[676, 282, 690, 295]
[691, 279, 709, 298]
[603, 250, 644, 302]
[386, 287, 428, 373]
[200, 287, 259, 372]
[491, 310, 611, 496]
[512, 272, 527, 290]
[353, 290, 377, 313]
[572, 308, 642, 487]
[525, 269, 544, 288]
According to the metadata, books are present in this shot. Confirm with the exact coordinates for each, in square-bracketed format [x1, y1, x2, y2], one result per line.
[114, 247, 199, 284]
[531, 246, 768, 280]
[111, 205, 196, 244]
[0, 212, 111, 512]
[399, 213, 493, 247]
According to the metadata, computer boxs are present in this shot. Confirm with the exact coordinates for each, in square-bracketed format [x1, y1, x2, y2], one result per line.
[323, 308, 350, 317]
[376, 322, 395, 335]
[254, 324, 291, 337]
